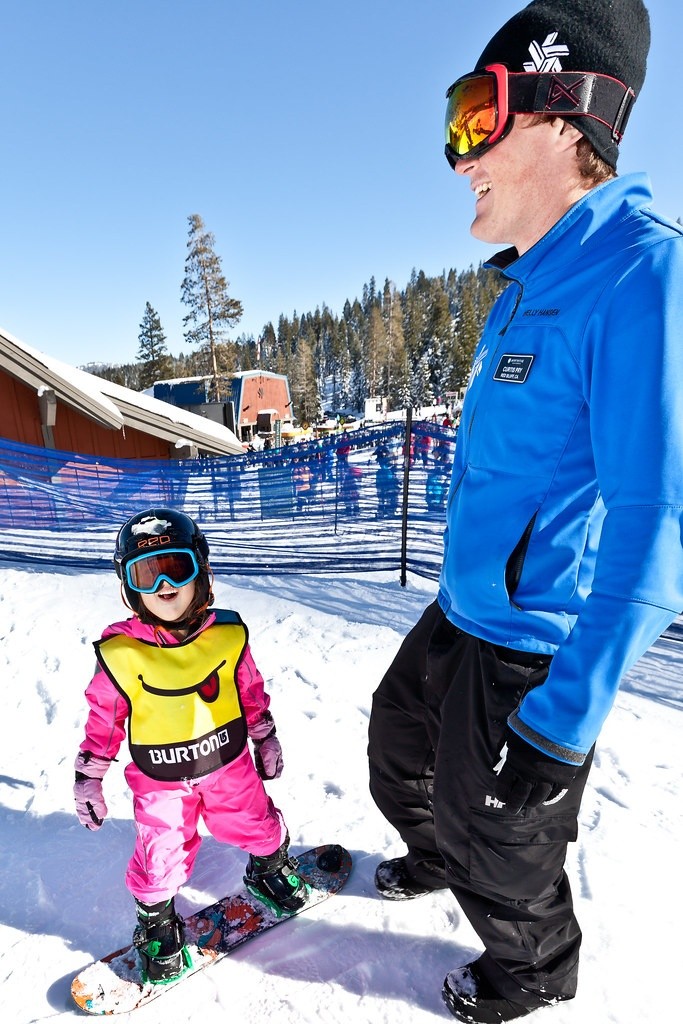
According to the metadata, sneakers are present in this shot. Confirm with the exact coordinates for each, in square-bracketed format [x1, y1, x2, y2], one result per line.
[441, 956, 577, 1024]
[132, 916, 188, 982]
[371, 854, 432, 901]
[244, 854, 308, 910]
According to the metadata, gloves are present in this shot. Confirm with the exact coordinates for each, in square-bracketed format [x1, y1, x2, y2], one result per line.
[254, 719, 284, 778]
[73, 752, 112, 831]
[490, 726, 582, 814]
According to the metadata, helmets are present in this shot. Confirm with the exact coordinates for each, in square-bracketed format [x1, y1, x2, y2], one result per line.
[114, 508, 208, 581]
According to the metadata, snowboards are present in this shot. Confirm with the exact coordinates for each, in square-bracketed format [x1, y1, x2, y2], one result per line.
[70, 843, 352, 1015]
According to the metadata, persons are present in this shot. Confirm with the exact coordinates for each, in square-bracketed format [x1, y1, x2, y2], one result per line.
[353, 1, 683, 1024]
[284, 412, 459, 519]
[72, 506, 305, 987]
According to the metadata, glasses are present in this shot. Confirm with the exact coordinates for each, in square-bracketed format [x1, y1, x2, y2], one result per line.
[444, 67, 630, 164]
[120, 543, 199, 597]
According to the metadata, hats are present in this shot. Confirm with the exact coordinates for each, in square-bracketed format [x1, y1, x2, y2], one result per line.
[475, 1, 652, 163]
